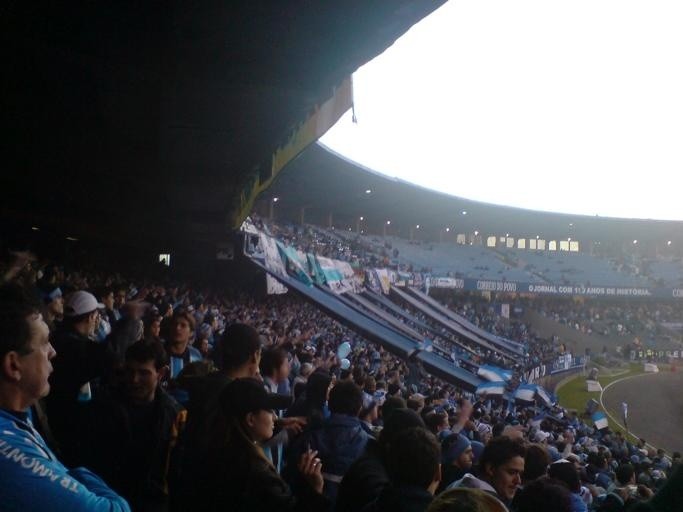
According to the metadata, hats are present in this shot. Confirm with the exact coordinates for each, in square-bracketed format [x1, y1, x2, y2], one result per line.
[218, 377, 293, 415]
[534, 431, 550, 442]
[409, 393, 429, 402]
[441, 433, 471, 465]
[63, 290, 106, 318]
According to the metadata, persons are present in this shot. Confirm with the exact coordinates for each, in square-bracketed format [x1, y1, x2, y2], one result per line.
[1, 248, 525, 510]
[525, 295, 683, 366]
[525, 392, 683, 511]
[250, 210, 390, 247]
